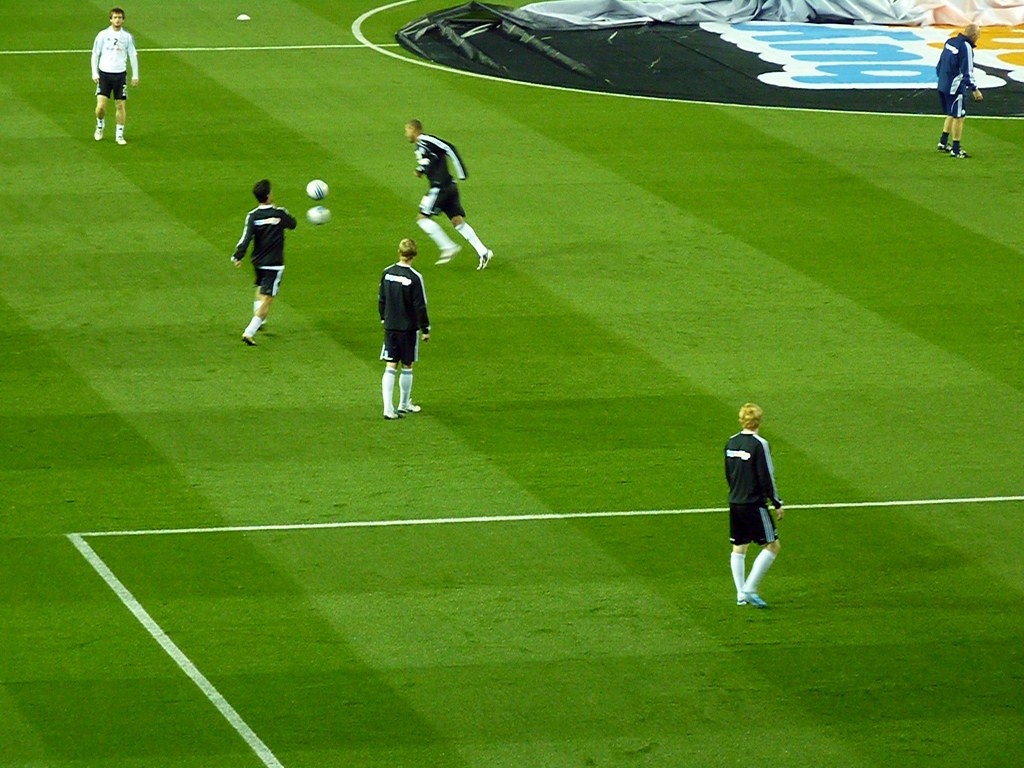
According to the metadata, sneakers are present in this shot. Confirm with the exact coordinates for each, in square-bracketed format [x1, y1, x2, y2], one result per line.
[737, 595, 747, 605]
[383, 409, 405, 419]
[434, 247, 460, 265]
[738, 591, 767, 608]
[951, 149, 968, 159]
[476, 250, 492, 270]
[937, 143, 954, 153]
[398, 398, 421, 413]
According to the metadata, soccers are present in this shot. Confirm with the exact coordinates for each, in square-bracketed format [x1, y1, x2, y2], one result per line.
[307, 205, 330, 225]
[306, 179, 329, 200]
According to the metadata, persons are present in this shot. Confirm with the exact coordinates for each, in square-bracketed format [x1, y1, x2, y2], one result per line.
[231, 180, 298, 346]
[725, 403, 785, 608]
[405, 120, 494, 272]
[91, 8, 139, 145]
[936, 24, 983, 159]
[379, 239, 431, 419]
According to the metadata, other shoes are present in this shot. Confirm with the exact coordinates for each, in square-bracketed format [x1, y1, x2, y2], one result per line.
[242, 333, 256, 346]
[94, 123, 104, 141]
[116, 136, 126, 145]
